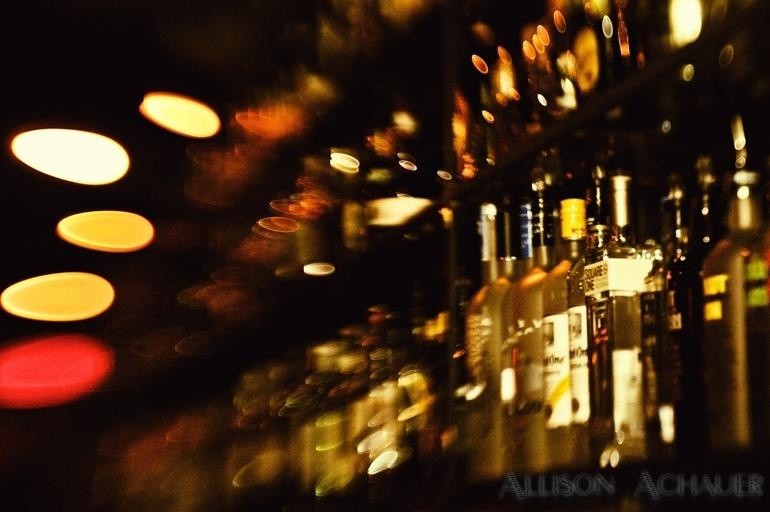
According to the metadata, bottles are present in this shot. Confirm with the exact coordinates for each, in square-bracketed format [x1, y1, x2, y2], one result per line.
[460, 146, 769, 471]
